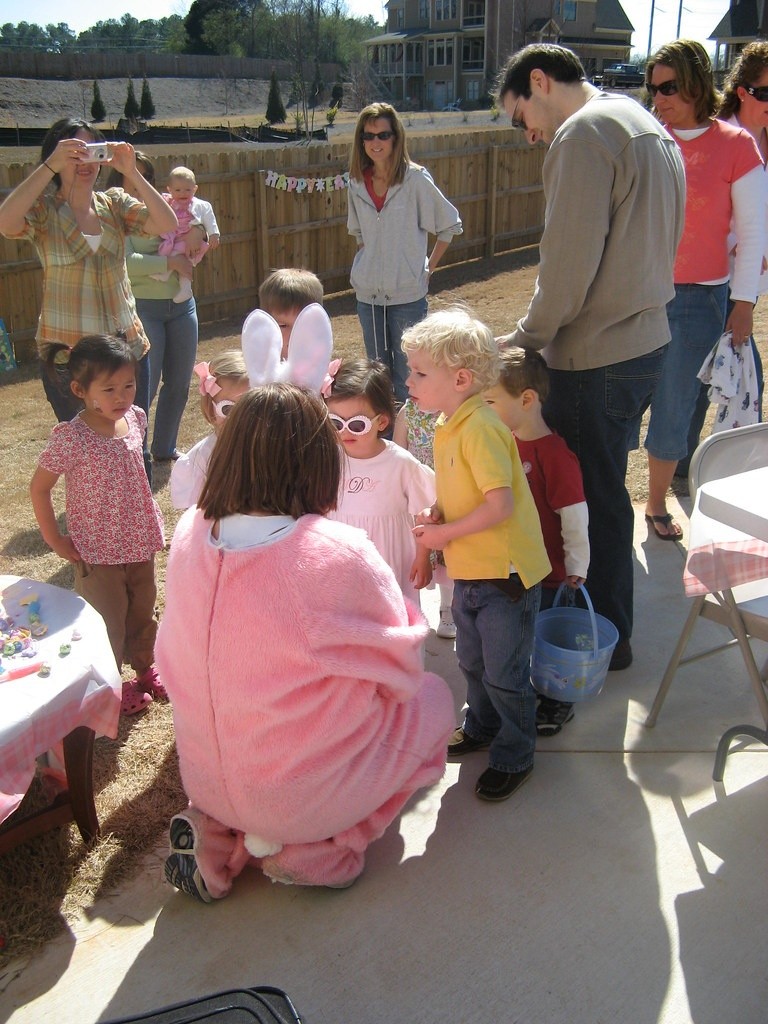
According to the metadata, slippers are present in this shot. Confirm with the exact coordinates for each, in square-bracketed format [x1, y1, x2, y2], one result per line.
[118, 669, 166, 715]
[645, 511, 682, 540]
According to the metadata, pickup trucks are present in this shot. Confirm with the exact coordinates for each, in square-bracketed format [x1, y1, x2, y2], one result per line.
[592, 64, 646, 88]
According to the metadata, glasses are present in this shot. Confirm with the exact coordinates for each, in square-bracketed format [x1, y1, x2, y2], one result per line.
[511, 95, 522, 128]
[360, 131, 396, 140]
[646, 80, 685, 97]
[327, 413, 383, 437]
[211, 400, 235, 420]
[143, 174, 155, 183]
[740, 79, 768, 102]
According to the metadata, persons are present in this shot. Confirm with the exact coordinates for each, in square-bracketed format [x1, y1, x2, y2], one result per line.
[628, 39, 768, 542]
[494, 43, 687, 671]
[30, 335, 168, 716]
[169, 268, 438, 640]
[0, 117, 221, 492]
[400, 303, 590, 801]
[153, 382, 456, 903]
[348, 102, 464, 405]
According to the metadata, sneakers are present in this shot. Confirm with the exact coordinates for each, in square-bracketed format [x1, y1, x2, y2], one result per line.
[530, 683, 575, 737]
[607, 636, 632, 672]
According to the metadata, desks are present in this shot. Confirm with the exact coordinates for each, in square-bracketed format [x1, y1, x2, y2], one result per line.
[0, 573, 122, 850]
[681, 470, 767, 783]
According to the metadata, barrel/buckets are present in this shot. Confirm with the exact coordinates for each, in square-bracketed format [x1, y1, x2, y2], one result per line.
[529, 575, 618, 704]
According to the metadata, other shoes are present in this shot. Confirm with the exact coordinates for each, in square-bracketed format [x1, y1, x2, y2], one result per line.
[153, 449, 187, 461]
[163, 807, 211, 905]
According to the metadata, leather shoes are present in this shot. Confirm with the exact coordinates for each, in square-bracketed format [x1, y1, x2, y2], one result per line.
[475, 762, 535, 802]
[447, 726, 492, 756]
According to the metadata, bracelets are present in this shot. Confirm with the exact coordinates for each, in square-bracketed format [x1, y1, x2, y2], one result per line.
[43, 162, 56, 174]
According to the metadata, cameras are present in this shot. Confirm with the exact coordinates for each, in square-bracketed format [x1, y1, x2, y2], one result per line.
[79, 143, 108, 163]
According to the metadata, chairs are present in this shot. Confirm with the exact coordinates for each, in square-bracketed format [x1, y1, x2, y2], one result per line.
[645, 420, 767, 734]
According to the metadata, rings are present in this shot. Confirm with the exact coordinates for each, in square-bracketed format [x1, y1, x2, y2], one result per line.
[744, 336, 749, 338]
[196, 251, 200, 253]
